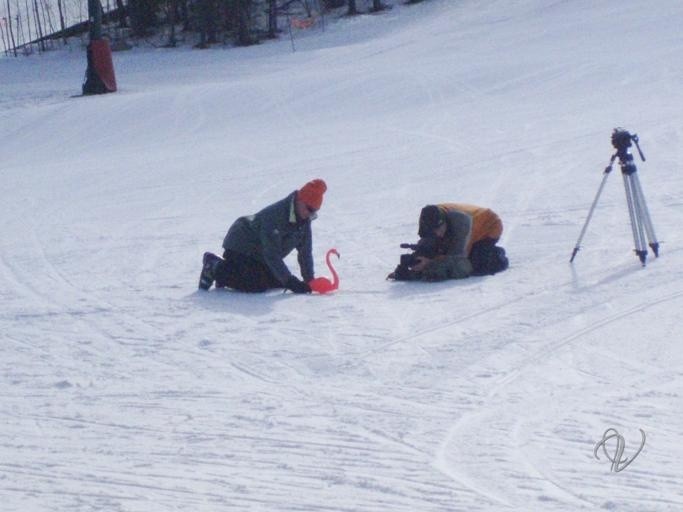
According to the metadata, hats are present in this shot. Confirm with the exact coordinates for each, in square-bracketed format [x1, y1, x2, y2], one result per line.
[298, 179, 327, 210]
[418, 206, 445, 236]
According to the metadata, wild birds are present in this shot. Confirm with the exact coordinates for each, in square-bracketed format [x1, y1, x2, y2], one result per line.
[282, 248, 341, 298]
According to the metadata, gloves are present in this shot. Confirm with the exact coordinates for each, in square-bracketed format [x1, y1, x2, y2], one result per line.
[285, 276, 311, 293]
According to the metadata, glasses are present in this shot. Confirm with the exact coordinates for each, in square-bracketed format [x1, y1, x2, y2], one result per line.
[305, 203, 316, 214]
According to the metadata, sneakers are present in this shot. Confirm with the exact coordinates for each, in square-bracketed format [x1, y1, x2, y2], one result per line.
[200, 254, 226, 288]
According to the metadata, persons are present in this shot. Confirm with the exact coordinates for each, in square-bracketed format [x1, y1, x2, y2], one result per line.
[197, 179, 327, 296]
[410, 200, 512, 278]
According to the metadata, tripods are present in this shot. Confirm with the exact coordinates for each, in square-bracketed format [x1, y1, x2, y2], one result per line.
[568, 126, 659, 267]
